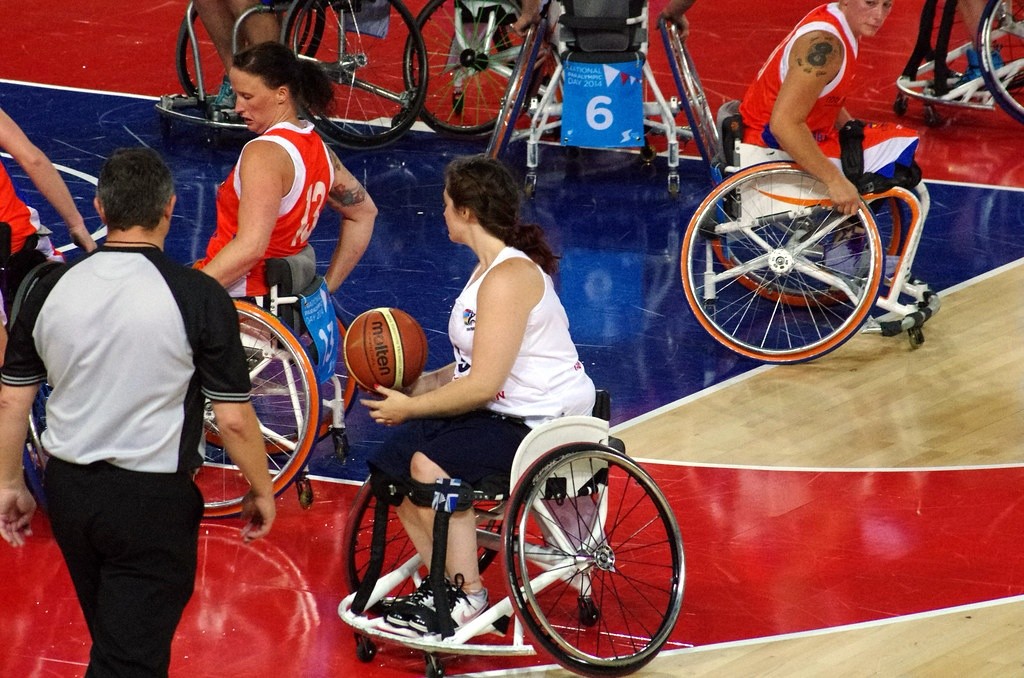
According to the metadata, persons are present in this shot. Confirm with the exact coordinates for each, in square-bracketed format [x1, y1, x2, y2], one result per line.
[358, 152, 600, 635]
[732, 0, 923, 290]
[949, 0, 1006, 92]
[0, 109, 98, 371]
[508, 0, 698, 43]
[0, 144, 275, 678]
[188, 38, 379, 297]
[193, 0, 281, 109]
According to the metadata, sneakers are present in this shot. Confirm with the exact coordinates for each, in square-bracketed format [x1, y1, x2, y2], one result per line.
[409, 574, 490, 632]
[214, 75, 235, 109]
[824, 240, 901, 279]
[386, 575, 451, 626]
[953, 40, 1008, 100]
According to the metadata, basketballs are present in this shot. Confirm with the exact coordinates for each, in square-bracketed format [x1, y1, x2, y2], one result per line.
[342, 307, 427, 397]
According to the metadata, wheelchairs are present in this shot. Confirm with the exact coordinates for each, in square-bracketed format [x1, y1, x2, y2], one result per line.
[337, 390, 687, 677]
[402, 0, 553, 143]
[676, 101, 942, 368]
[153, 1, 430, 153]
[26, 244, 360, 523]
[892, 0, 1023, 137]
[482, 0, 727, 191]
